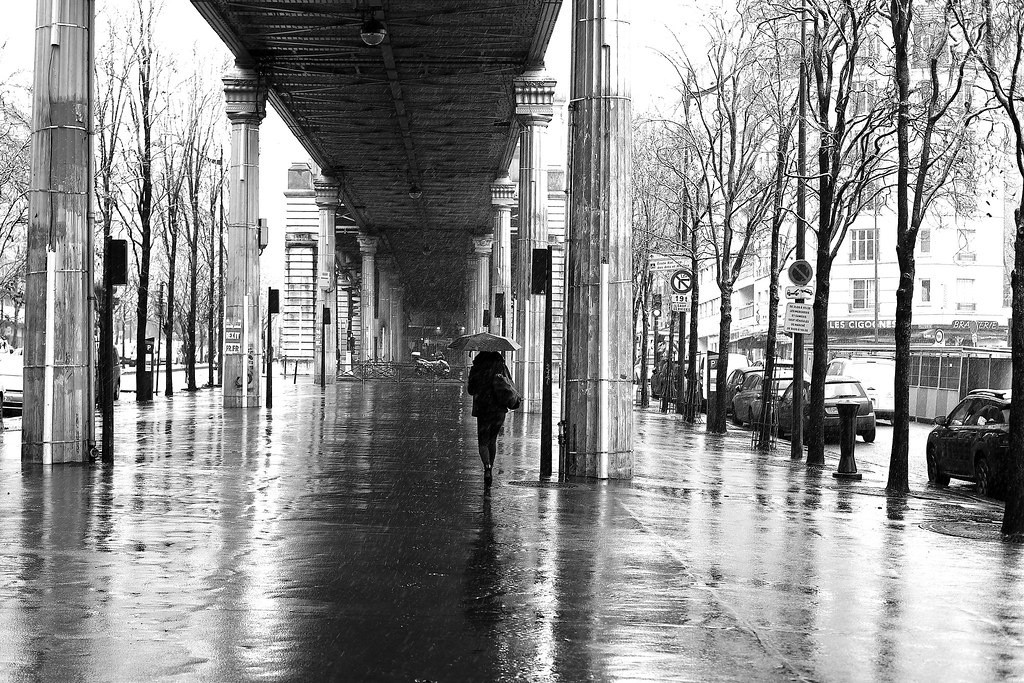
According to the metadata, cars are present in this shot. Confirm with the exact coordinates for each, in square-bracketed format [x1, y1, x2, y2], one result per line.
[773, 373, 877, 444]
[730, 369, 811, 430]
[651, 355, 754, 414]
[726, 367, 766, 416]
[93, 344, 123, 403]
[213, 352, 220, 370]
[0, 351, 23, 414]
[750, 358, 794, 371]
[633, 355, 655, 385]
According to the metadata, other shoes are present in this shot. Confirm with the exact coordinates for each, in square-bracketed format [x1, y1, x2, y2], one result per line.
[485, 464, 492, 480]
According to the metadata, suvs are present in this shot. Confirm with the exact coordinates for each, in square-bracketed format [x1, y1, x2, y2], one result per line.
[927, 387, 1013, 500]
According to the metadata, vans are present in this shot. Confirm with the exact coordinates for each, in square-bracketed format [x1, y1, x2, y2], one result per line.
[825, 355, 896, 425]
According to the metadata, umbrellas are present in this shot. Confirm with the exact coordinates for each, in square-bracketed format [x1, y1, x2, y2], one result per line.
[446, 332, 523, 352]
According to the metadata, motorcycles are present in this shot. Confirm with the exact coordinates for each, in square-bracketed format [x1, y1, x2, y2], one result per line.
[412, 351, 451, 379]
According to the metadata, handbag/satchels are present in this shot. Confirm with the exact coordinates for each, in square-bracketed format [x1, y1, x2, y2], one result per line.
[492, 363, 521, 410]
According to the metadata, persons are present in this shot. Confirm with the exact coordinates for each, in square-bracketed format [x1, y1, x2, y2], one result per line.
[467, 351, 512, 483]
